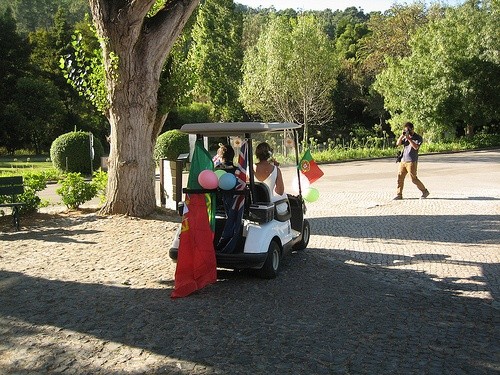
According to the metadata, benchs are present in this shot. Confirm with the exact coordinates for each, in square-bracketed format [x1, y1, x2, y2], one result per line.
[0, 175, 27, 232]
[217, 182, 292, 222]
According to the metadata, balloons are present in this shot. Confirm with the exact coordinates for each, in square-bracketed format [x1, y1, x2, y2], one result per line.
[198, 170, 236, 191]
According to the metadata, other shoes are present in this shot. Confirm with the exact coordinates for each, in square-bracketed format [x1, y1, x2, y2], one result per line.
[393, 194, 402, 200]
[291, 228, 301, 238]
[421, 189, 429, 197]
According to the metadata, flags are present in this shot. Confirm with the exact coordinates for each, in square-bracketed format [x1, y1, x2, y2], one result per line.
[215, 143, 247, 254]
[297, 149, 325, 185]
[170, 140, 217, 298]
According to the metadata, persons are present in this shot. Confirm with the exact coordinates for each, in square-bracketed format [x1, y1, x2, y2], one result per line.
[212, 144, 240, 178]
[393, 122, 429, 200]
[246, 142, 288, 214]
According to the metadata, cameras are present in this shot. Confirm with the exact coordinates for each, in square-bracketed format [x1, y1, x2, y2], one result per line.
[403, 130, 410, 136]
